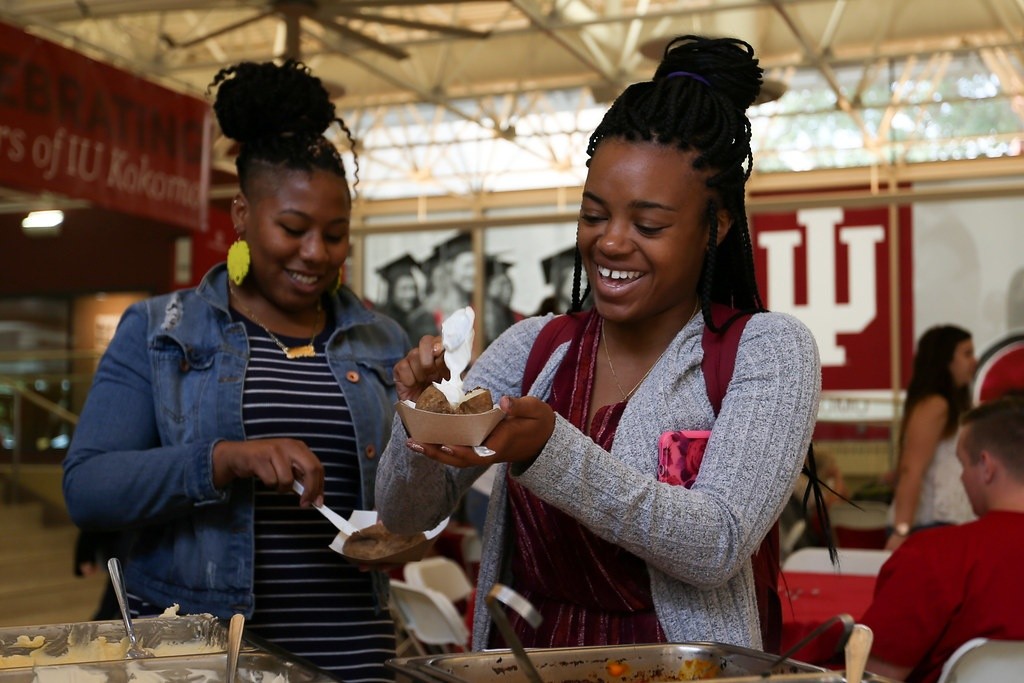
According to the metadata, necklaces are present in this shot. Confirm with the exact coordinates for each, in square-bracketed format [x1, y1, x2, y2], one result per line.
[229, 287, 320, 359]
[601, 302, 697, 401]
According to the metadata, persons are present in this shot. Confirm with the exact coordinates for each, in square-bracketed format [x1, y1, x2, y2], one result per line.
[885, 324, 980, 551]
[534, 247, 589, 317]
[819, 385, 1024, 683]
[61, 55, 414, 683]
[373, 35, 824, 650]
[375, 230, 516, 363]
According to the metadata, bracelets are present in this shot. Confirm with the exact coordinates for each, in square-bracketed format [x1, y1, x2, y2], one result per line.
[894, 522, 910, 536]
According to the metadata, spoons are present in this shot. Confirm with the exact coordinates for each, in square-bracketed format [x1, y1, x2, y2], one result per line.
[107, 557, 161, 671]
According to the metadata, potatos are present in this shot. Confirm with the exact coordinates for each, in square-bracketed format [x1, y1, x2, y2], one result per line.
[415, 374, 494, 415]
[343, 521, 428, 560]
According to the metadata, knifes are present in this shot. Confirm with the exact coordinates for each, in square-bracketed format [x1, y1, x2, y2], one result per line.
[290, 479, 359, 538]
